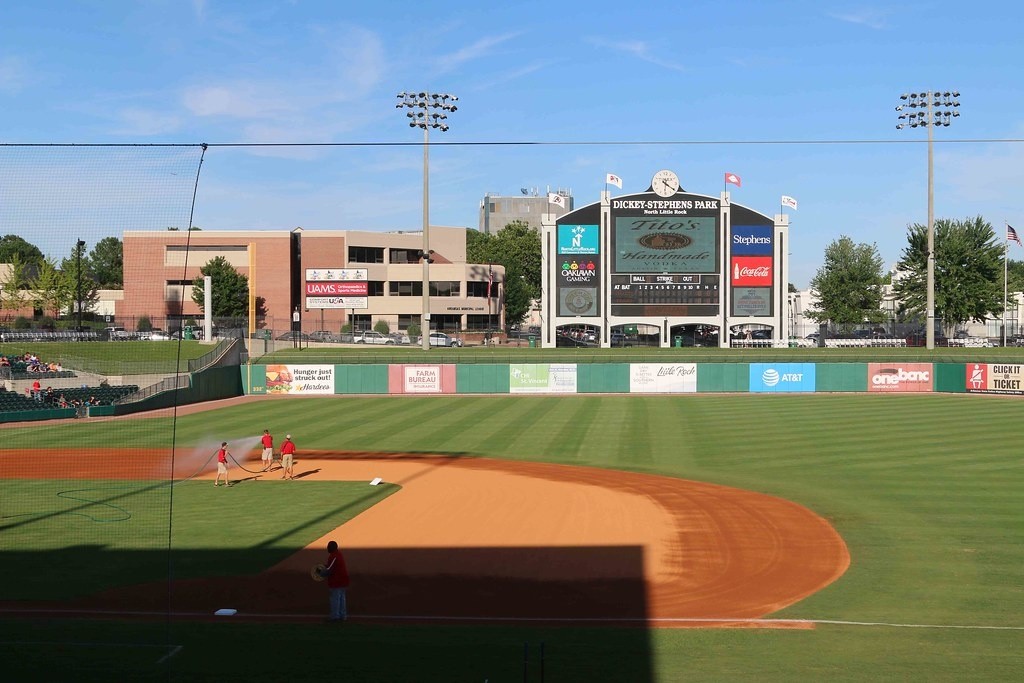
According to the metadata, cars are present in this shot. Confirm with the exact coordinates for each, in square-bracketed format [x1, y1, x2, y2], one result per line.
[805, 325, 1024, 347]
[417, 332, 462, 348]
[173, 326, 203, 340]
[103, 326, 126, 332]
[310, 330, 331, 342]
[274, 331, 310, 342]
[510, 325, 516, 330]
[529, 326, 628, 343]
[140, 331, 181, 341]
[354, 331, 397, 345]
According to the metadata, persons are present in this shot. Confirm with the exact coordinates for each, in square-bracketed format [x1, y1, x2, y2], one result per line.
[213, 441, 231, 486]
[43, 386, 55, 405]
[59, 392, 67, 409]
[24, 351, 63, 373]
[280, 434, 296, 481]
[70, 396, 101, 416]
[32, 378, 42, 404]
[261, 429, 274, 472]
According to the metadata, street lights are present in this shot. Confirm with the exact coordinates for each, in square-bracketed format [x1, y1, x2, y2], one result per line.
[76, 240, 85, 332]
[895, 89, 961, 346]
[394, 91, 460, 351]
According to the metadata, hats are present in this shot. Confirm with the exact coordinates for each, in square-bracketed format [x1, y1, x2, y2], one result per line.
[286, 435, 291, 438]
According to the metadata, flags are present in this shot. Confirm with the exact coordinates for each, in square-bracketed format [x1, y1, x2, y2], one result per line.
[1007, 224, 1023, 247]
[781, 196, 797, 211]
[606, 173, 623, 190]
[549, 193, 566, 209]
[725, 173, 741, 187]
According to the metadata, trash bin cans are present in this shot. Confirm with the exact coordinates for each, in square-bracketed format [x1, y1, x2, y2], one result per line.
[264, 329, 272, 340]
[528, 336, 536, 348]
[184, 326, 192, 341]
[675, 336, 683, 347]
[789, 336, 797, 348]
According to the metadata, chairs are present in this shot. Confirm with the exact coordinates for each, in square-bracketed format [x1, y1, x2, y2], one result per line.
[0, 354, 138, 411]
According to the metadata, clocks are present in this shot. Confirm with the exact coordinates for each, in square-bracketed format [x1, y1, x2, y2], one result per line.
[652, 169, 679, 198]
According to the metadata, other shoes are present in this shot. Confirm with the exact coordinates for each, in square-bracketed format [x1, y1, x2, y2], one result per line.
[290, 476, 293, 480]
[341, 616, 346, 621]
[281, 476, 285, 478]
[328, 617, 339, 621]
[225, 484, 231, 487]
[214, 483, 218, 486]
